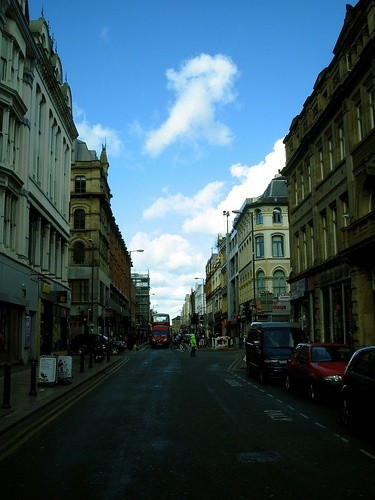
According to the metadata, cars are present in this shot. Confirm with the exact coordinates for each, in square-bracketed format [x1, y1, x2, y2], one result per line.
[340, 346, 375, 435]
[283, 342, 359, 402]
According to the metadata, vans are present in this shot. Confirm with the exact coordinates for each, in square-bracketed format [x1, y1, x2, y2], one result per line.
[245, 321, 307, 384]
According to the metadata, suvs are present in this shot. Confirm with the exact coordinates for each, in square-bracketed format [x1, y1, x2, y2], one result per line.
[70, 333, 128, 356]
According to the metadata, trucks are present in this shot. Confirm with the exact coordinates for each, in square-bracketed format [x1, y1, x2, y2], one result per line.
[148, 321, 172, 348]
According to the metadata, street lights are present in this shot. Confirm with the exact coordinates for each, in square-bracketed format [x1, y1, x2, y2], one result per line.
[195, 277, 204, 314]
[148, 293, 155, 320]
[232, 209, 257, 321]
[129, 249, 145, 334]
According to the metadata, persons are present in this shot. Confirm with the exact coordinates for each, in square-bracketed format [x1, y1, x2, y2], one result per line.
[314, 308, 321, 343]
[126, 333, 135, 352]
[191, 335, 197, 357]
[175, 333, 186, 348]
[92, 334, 105, 361]
